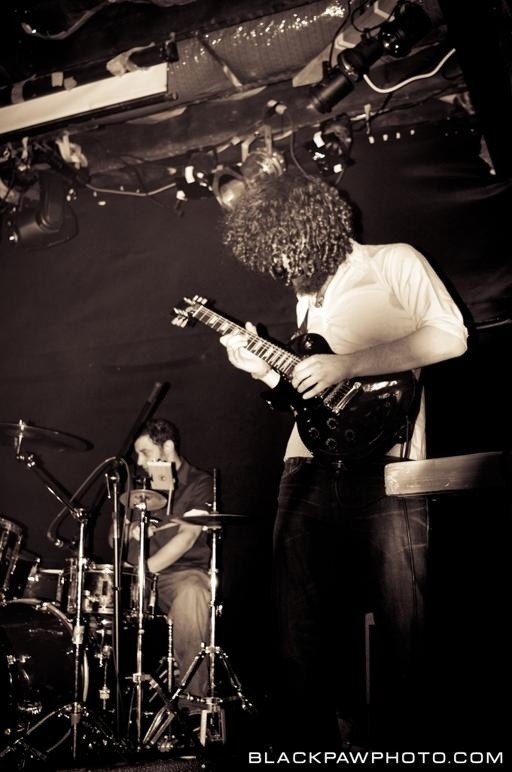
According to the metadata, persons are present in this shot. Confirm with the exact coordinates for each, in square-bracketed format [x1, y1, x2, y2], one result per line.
[218, 165, 472, 762]
[108, 418, 219, 760]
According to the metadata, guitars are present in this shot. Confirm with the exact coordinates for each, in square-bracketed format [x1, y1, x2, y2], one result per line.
[170, 293, 420, 467]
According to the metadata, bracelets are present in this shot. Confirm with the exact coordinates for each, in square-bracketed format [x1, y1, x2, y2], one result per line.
[250, 364, 273, 380]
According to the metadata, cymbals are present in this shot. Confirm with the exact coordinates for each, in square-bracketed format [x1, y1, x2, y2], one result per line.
[168, 510, 249, 530]
[119, 489, 166, 512]
[3, 423, 89, 454]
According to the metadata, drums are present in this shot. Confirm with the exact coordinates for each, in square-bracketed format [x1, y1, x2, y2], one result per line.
[0, 517, 22, 601]
[0, 599, 92, 753]
[80, 564, 157, 620]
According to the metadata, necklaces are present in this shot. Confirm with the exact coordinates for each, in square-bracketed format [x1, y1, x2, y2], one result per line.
[315, 270, 332, 308]
